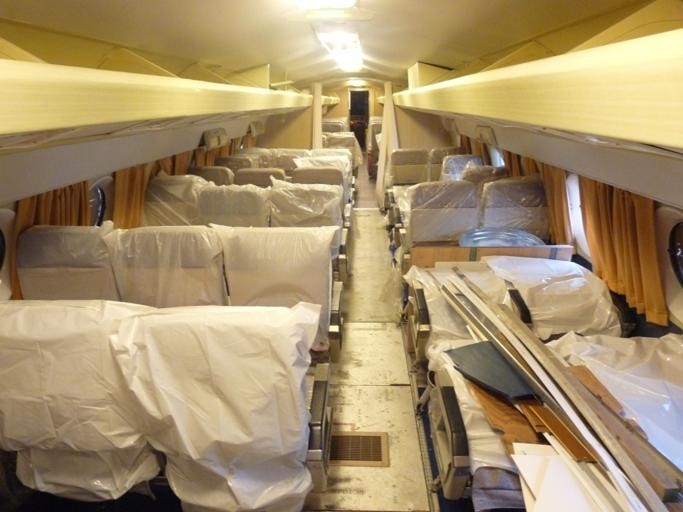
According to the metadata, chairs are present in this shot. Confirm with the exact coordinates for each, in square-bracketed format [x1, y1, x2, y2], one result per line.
[380, 141, 557, 253]
